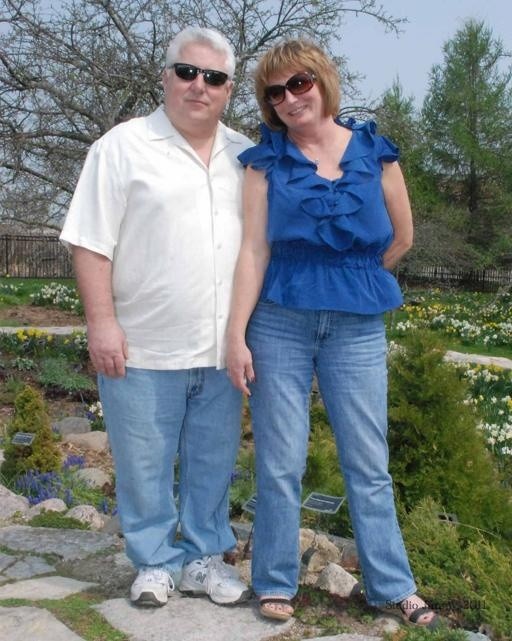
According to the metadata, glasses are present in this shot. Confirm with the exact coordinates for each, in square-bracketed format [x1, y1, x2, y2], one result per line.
[168, 63, 229, 87]
[262, 71, 317, 108]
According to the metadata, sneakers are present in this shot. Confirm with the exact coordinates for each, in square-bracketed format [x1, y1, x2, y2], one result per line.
[177, 556, 254, 606]
[130, 565, 174, 608]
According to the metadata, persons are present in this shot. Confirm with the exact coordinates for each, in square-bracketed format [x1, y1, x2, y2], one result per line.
[59, 27, 437, 625]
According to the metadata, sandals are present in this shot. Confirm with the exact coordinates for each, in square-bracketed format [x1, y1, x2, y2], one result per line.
[258, 592, 295, 621]
[377, 596, 437, 629]
